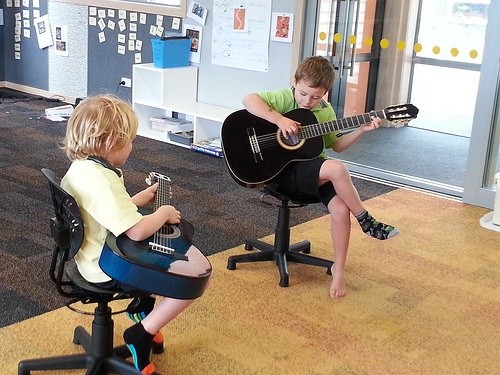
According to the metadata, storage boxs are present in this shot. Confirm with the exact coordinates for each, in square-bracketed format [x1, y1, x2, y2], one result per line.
[151, 37, 193, 69]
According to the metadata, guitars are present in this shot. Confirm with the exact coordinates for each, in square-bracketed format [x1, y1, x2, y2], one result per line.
[98, 171, 212, 300]
[221, 103, 420, 189]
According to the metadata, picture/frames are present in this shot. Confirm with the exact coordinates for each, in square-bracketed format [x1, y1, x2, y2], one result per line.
[271, 12, 294, 42]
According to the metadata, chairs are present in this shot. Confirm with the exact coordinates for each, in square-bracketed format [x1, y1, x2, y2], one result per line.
[227, 182, 334, 287]
[18, 168, 151, 374]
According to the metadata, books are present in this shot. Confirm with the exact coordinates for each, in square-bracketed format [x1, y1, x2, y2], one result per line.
[190, 141, 223, 156]
[150, 116, 193, 132]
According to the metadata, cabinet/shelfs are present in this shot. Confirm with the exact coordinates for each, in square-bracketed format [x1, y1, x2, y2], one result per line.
[131, 63, 239, 156]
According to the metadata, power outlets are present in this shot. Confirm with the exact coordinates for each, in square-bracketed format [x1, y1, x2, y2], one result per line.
[120, 77, 131, 88]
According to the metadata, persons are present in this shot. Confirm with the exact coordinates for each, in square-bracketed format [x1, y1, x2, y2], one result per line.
[60, 94, 211, 375]
[242, 55, 399, 298]
